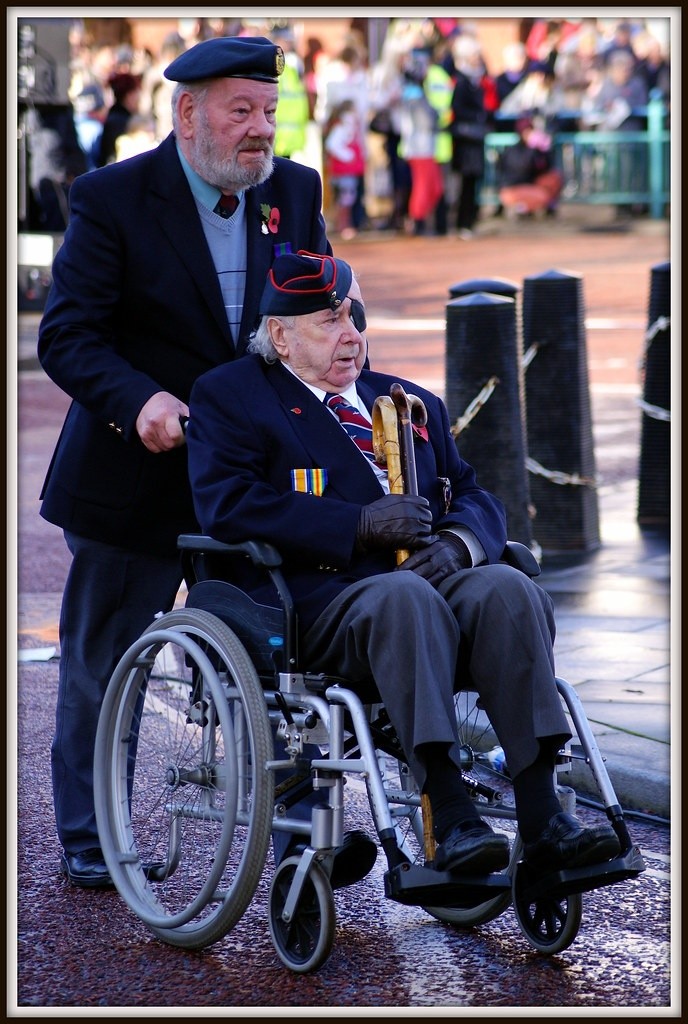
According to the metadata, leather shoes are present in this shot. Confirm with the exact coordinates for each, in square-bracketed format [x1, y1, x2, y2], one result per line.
[279, 828, 378, 891]
[434, 818, 510, 872]
[523, 811, 620, 872]
[60, 851, 112, 886]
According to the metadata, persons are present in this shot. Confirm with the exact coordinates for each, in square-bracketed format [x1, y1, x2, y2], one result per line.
[187, 250, 625, 878]
[32, 37, 376, 894]
[69, 16, 671, 243]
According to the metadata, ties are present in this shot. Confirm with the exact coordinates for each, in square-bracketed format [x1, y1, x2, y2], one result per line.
[215, 195, 239, 219]
[324, 393, 388, 476]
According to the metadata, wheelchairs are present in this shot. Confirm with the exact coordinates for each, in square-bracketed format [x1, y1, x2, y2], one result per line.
[90, 412, 646, 973]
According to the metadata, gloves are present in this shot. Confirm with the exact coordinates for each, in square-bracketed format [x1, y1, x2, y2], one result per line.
[397, 531, 472, 584]
[357, 494, 440, 549]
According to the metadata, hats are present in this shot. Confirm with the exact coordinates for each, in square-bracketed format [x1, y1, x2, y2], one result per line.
[163, 36, 285, 84]
[259, 249, 352, 316]
